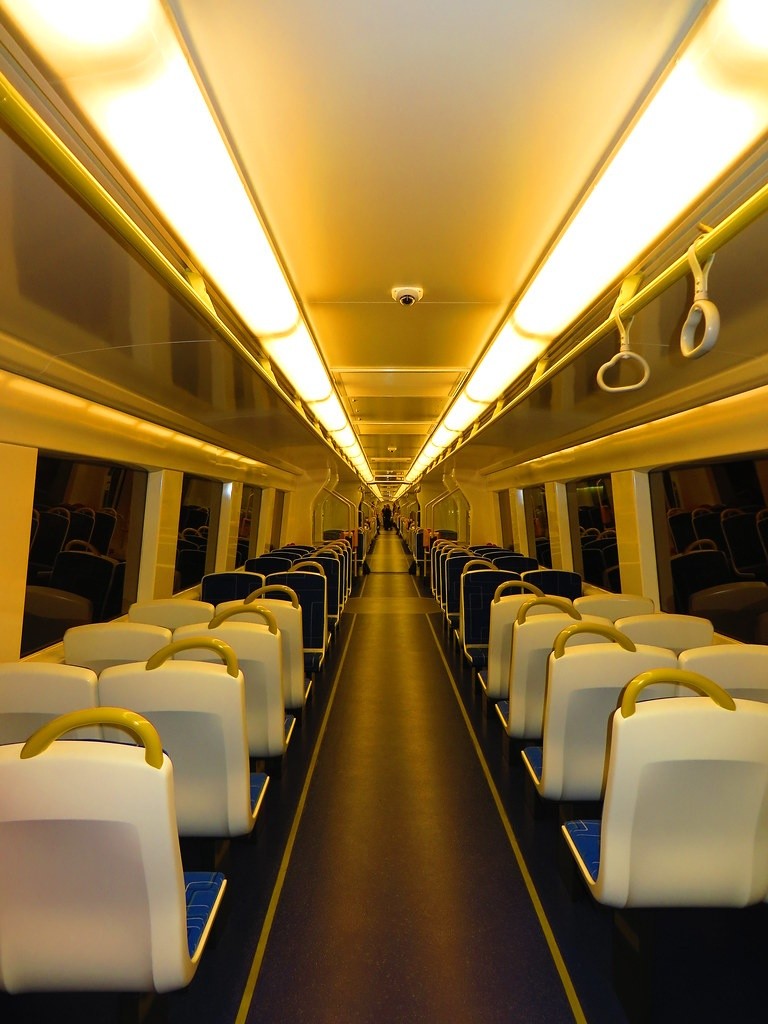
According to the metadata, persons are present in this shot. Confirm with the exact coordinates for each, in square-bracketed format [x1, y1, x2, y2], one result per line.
[384, 504, 393, 531]
[382, 504, 388, 529]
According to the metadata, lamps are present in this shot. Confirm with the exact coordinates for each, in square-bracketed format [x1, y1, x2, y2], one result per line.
[0, 2, 381, 503]
[391, 0, 768, 500]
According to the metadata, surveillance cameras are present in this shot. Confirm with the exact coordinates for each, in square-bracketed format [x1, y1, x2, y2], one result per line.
[392, 288, 423, 306]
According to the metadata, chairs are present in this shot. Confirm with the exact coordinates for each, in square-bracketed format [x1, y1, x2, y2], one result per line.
[244, 557, 292, 578]
[520, 569, 583, 604]
[21, 501, 249, 659]
[128, 599, 214, 636]
[175, 607, 298, 790]
[214, 584, 314, 738]
[477, 583, 572, 736]
[264, 561, 327, 698]
[677, 644, 768, 705]
[260, 512, 377, 618]
[572, 593, 655, 625]
[0, 661, 102, 745]
[99, 636, 270, 874]
[393, 512, 540, 638]
[201, 572, 266, 608]
[494, 598, 615, 782]
[458, 559, 524, 702]
[561, 667, 768, 955]
[520, 622, 677, 835]
[614, 613, 714, 659]
[293, 549, 341, 648]
[536, 502, 768, 645]
[0, 707, 226, 1023]
[63, 622, 172, 682]
